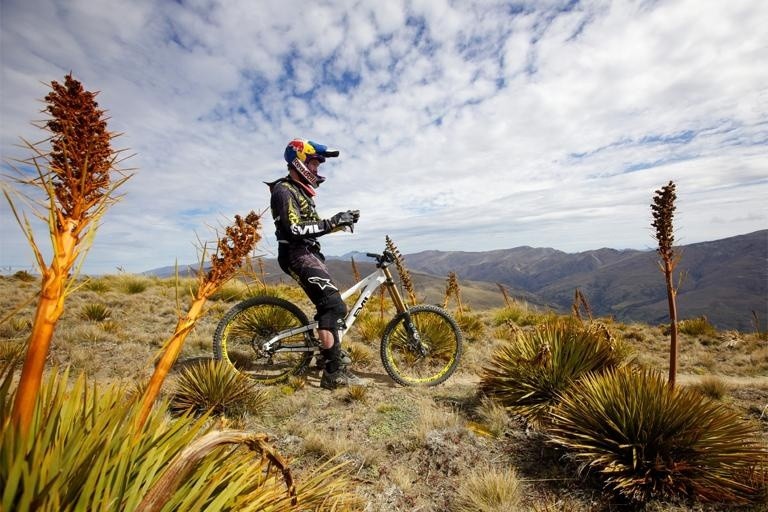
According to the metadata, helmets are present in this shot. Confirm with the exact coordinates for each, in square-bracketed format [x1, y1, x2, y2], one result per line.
[284, 139, 340, 188]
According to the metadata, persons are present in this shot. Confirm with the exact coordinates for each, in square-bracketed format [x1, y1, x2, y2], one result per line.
[263, 138, 369, 390]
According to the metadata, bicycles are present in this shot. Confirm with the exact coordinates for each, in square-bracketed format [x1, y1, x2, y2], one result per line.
[211, 249, 463, 390]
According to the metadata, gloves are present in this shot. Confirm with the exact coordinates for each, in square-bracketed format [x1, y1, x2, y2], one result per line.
[332, 209, 358, 225]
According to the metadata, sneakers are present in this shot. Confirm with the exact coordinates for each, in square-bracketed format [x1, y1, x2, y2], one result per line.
[319, 366, 375, 389]
